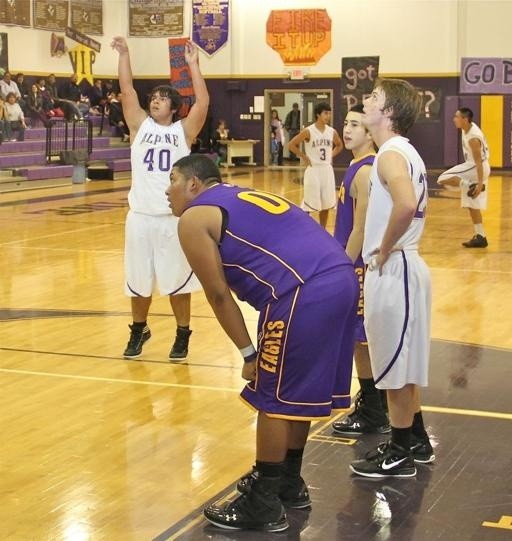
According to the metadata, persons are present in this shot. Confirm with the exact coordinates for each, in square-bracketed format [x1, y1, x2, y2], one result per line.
[329, 105, 391, 437]
[16, 73, 31, 96]
[437, 107, 492, 248]
[28, 84, 56, 129]
[283, 103, 301, 163]
[210, 120, 235, 167]
[46, 74, 58, 99]
[0, 72, 21, 104]
[106, 94, 131, 142]
[84, 78, 103, 106]
[164, 153, 360, 533]
[109, 35, 211, 362]
[3, 92, 27, 140]
[38, 76, 61, 114]
[349, 79, 436, 480]
[65, 73, 89, 119]
[286, 102, 344, 228]
[270, 109, 284, 165]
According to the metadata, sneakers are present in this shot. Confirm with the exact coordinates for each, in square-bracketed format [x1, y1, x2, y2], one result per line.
[467, 183, 486, 196]
[378, 440, 435, 464]
[351, 440, 417, 479]
[123, 323, 151, 358]
[169, 329, 191, 360]
[238, 470, 313, 508]
[203, 494, 289, 533]
[462, 238, 487, 248]
[332, 410, 391, 434]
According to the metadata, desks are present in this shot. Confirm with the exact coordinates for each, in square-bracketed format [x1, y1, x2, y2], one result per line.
[216, 138, 260, 168]
